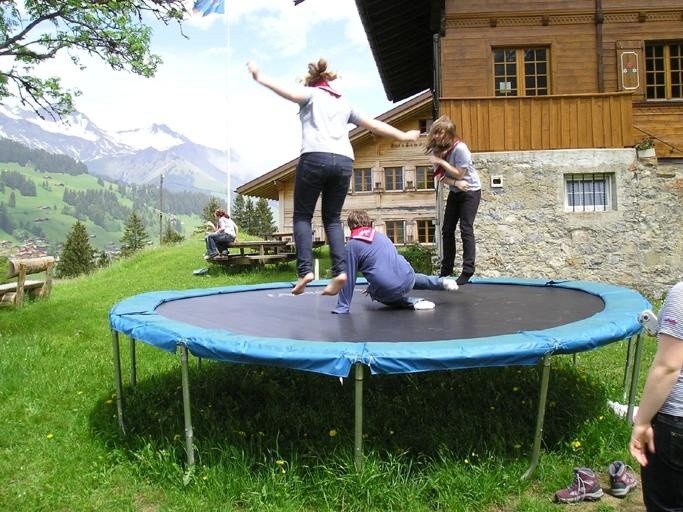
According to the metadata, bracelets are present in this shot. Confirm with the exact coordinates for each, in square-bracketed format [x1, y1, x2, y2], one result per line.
[453, 180, 457, 187]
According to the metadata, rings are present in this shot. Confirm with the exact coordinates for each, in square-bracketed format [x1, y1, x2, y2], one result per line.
[464, 188, 467, 190]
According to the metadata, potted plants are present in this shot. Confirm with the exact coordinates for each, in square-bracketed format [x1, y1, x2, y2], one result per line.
[635, 136, 657, 158]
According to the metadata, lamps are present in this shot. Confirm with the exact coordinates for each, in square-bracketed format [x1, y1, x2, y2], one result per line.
[637, 11, 648, 22]
[489, 16, 497, 27]
[539, 13, 552, 26]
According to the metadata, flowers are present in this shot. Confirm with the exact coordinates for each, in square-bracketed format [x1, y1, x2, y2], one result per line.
[623, 63, 637, 79]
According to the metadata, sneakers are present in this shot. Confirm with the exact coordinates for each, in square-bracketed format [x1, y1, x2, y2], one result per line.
[608, 459, 638, 501]
[553, 466, 604, 505]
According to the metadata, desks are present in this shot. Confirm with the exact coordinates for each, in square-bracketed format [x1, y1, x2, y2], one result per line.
[227, 240, 289, 272]
[262, 229, 315, 253]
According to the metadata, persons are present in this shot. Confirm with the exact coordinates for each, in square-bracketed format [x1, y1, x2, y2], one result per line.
[245, 58, 423, 298]
[206, 214, 238, 257]
[203, 209, 234, 260]
[626, 275, 681, 512]
[329, 209, 461, 315]
[424, 112, 483, 286]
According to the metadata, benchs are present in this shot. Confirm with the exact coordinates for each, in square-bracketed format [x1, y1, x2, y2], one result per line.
[293, 239, 325, 252]
[0, 256, 55, 307]
[212, 251, 296, 271]
[252, 247, 271, 253]
